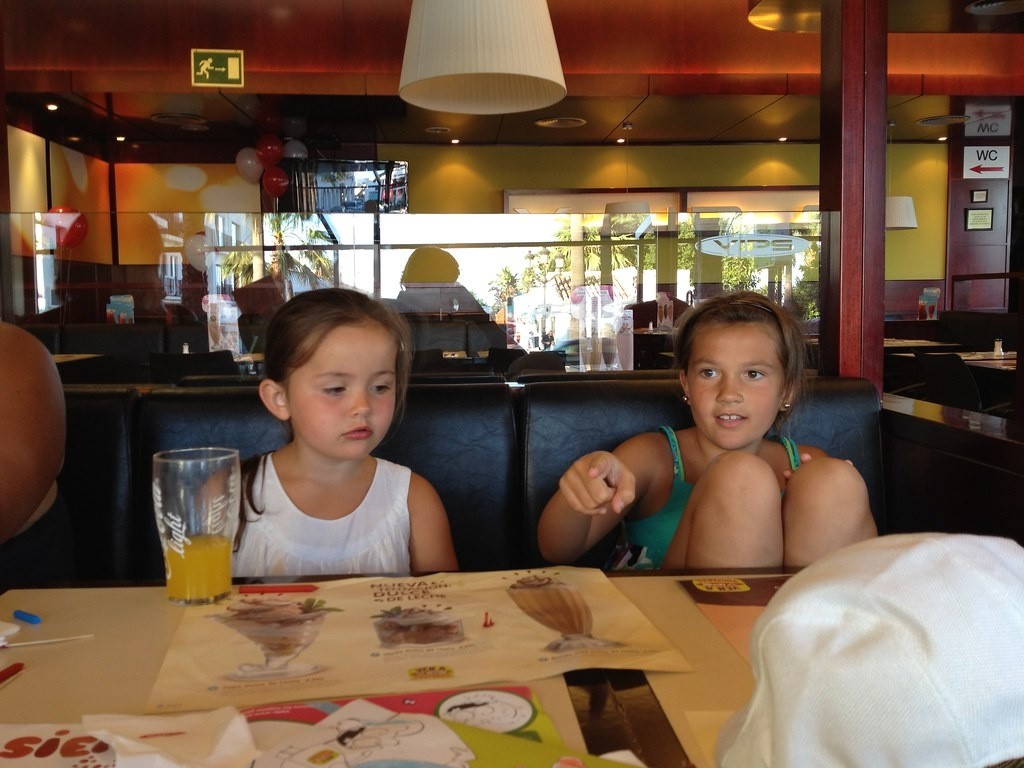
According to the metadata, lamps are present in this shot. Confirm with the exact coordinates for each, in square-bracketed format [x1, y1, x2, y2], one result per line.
[604, 122, 653, 235]
[398, 0, 568, 116]
[881, 122, 920, 231]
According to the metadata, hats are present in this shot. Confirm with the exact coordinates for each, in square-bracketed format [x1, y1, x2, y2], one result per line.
[710, 533, 1024, 768]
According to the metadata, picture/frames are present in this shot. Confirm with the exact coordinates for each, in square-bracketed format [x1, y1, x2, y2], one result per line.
[967, 207, 994, 232]
[971, 187, 988, 203]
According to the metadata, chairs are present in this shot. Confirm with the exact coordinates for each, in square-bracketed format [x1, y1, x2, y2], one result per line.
[0, 304, 881, 587]
[912, 351, 1015, 417]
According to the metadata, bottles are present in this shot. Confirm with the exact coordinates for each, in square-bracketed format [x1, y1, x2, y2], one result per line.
[649, 321, 654, 331]
[994, 339, 1004, 358]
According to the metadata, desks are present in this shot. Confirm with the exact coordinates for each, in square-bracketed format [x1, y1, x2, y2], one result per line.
[804, 337, 959, 384]
[234, 349, 492, 363]
[0, 561, 804, 768]
[49, 353, 101, 365]
[891, 349, 1019, 411]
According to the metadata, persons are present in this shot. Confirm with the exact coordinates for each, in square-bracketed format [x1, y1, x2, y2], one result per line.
[201, 288, 457, 579]
[713, 533, 1024, 768]
[538, 291, 877, 567]
[0, 320, 88, 594]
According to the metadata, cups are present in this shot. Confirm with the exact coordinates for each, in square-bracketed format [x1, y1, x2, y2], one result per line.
[154, 447, 241, 605]
